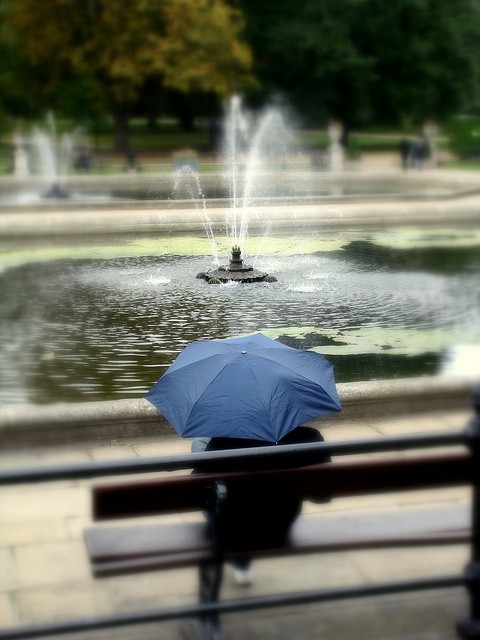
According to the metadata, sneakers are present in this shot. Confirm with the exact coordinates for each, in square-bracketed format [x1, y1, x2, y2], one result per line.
[234, 570, 254, 588]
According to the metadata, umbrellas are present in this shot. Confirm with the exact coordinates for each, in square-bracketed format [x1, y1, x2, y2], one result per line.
[144, 333, 344, 445]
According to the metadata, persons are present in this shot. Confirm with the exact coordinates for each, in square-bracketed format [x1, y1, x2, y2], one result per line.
[395, 139, 430, 171]
[191, 426, 332, 585]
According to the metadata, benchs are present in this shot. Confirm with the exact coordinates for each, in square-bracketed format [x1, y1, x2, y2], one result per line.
[83, 449, 479, 640]
